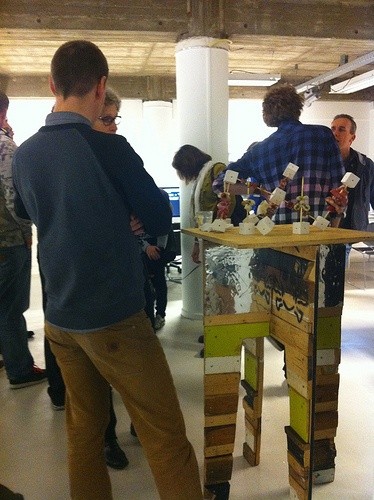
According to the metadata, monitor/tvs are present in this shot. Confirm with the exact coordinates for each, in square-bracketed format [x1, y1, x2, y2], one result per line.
[161, 186, 181, 217]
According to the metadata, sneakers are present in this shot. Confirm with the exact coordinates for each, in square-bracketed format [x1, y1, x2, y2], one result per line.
[153, 314, 166, 330]
[10, 364, 48, 388]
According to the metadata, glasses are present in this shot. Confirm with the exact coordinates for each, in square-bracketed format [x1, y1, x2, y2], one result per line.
[98, 115, 121, 126]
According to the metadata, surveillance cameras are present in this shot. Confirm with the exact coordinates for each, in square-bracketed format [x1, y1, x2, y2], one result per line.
[303, 90, 321, 108]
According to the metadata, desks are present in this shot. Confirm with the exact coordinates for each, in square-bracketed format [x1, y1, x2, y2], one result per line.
[182, 221, 373, 499]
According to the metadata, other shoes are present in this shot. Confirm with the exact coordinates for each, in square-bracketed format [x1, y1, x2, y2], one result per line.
[103, 433, 129, 469]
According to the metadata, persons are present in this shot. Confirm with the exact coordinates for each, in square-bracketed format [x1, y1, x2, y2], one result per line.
[8, 40, 203, 500]
[213, 85, 348, 379]
[0, 93, 50, 389]
[172, 145, 250, 359]
[330, 116, 374, 254]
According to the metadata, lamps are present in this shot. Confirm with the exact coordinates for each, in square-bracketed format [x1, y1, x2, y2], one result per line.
[294, 50, 374, 95]
[327, 69, 374, 95]
[228, 70, 281, 87]
